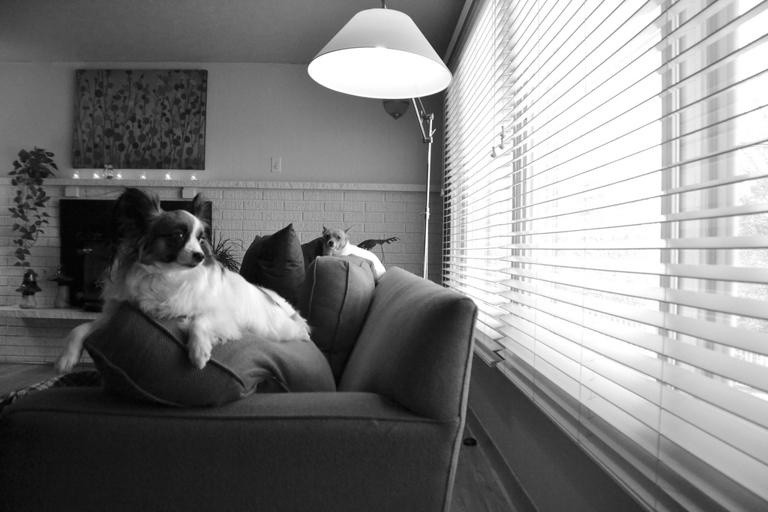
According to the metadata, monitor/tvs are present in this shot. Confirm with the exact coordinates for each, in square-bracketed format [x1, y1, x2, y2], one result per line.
[58, 198, 211, 312]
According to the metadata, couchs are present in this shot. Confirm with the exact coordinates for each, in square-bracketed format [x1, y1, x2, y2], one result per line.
[2, 227, 478, 511]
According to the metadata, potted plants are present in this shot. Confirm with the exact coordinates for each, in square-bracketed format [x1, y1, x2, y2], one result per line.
[6, 144, 60, 310]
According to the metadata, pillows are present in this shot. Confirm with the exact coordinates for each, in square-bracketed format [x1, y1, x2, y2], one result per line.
[87, 301, 338, 408]
[301, 253, 377, 378]
[238, 223, 307, 314]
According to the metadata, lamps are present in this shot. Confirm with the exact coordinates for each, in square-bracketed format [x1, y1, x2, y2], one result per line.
[307, 1, 453, 282]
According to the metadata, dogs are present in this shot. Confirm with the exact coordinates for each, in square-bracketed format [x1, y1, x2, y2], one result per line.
[52, 185, 313, 375]
[307, 223, 387, 281]
